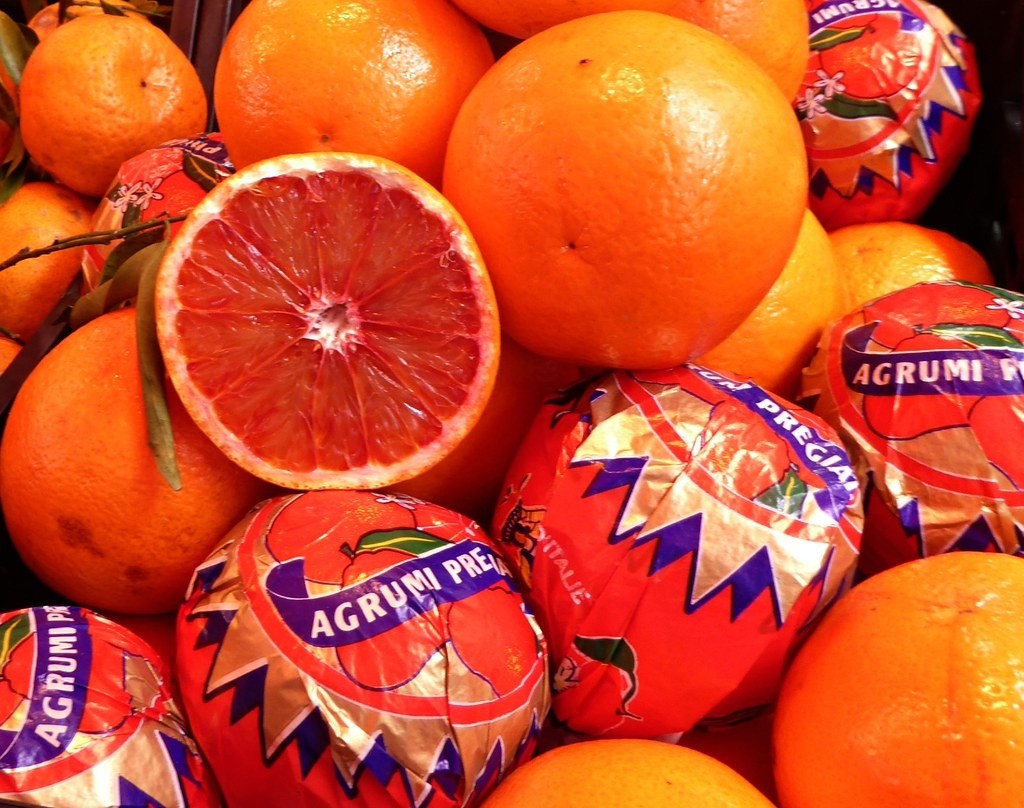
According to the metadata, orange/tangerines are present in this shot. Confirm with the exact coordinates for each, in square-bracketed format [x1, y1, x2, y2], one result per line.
[1, 0, 1024, 808]
